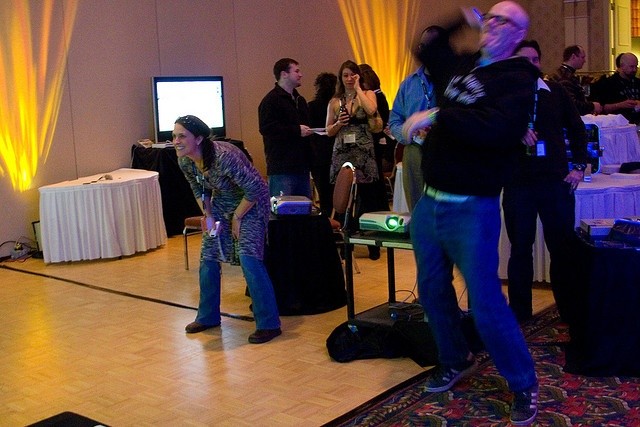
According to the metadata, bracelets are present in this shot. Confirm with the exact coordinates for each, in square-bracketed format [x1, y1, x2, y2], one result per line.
[232, 210, 241, 221]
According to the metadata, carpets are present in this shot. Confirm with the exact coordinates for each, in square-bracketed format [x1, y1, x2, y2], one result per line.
[319, 302, 640, 426]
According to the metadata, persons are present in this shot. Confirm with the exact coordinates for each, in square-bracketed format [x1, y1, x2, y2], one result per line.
[306, 72, 339, 218]
[595, 52, 640, 126]
[258, 57, 313, 198]
[388, 19, 471, 306]
[358, 64, 394, 260]
[502, 39, 589, 325]
[172, 115, 282, 344]
[330, 59, 379, 228]
[409, 2, 544, 421]
[548, 44, 604, 115]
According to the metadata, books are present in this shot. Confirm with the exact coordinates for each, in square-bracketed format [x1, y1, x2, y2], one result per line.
[582, 216, 640, 235]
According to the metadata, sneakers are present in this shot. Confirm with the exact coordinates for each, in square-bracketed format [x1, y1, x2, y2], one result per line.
[185, 322, 221, 334]
[248, 328, 282, 344]
[509, 372, 539, 426]
[423, 350, 478, 393]
[340, 244, 355, 260]
[367, 245, 380, 261]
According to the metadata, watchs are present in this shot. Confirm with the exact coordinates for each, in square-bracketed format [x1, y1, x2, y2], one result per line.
[575, 164, 586, 172]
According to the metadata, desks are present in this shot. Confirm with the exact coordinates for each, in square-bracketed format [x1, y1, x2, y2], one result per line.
[344, 231, 471, 320]
[599, 125, 639, 172]
[245, 210, 347, 316]
[130, 139, 253, 236]
[497, 172, 640, 283]
[39, 168, 167, 265]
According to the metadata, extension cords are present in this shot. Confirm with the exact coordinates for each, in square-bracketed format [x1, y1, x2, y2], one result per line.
[10, 247, 28, 259]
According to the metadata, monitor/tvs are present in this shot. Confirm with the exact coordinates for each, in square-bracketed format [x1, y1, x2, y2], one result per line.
[151, 73, 226, 144]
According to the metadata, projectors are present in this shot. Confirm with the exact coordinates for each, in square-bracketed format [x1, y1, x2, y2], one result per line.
[359, 211, 411, 234]
[270, 195, 313, 216]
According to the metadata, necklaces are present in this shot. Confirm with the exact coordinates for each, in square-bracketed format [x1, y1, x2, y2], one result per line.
[344, 91, 358, 97]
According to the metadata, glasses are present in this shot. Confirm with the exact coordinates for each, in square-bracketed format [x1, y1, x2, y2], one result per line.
[478, 14, 522, 31]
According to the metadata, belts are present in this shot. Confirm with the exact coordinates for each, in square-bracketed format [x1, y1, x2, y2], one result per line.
[423, 184, 475, 204]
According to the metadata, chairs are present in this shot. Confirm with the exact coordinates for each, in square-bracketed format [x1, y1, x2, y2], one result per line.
[328, 161, 361, 275]
[183, 216, 204, 270]
